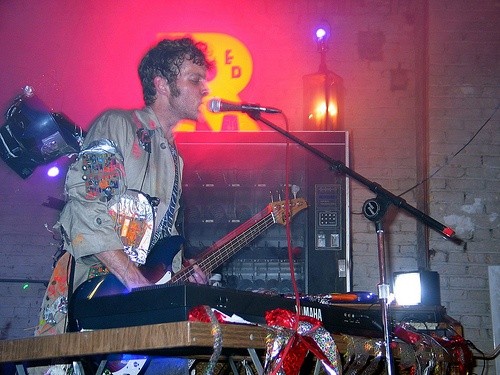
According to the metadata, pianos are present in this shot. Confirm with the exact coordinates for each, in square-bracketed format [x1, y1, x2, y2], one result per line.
[71, 276, 396, 340]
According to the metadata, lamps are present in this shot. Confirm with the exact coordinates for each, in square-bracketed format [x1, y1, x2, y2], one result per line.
[300, 20, 347, 133]
[0, 87, 84, 179]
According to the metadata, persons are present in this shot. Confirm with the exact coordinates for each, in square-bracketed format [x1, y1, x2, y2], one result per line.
[26, 37, 211, 375]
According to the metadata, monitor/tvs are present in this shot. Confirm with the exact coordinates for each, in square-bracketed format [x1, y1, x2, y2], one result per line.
[392, 271, 440, 307]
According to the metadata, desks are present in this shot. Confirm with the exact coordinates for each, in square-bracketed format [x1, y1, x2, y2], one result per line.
[0, 320, 472, 375]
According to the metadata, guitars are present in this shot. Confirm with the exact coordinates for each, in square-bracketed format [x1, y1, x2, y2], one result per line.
[66, 185, 310, 375]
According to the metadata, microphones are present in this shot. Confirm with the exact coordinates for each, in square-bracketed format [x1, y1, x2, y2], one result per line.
[206, 98, 282, 115]
